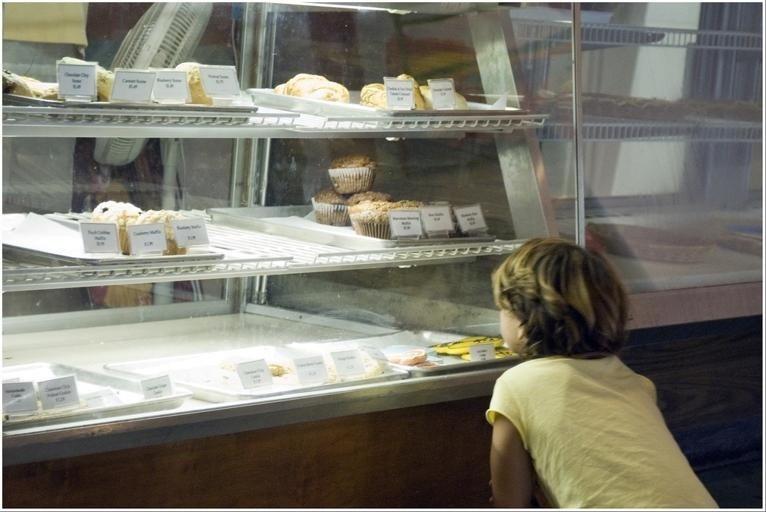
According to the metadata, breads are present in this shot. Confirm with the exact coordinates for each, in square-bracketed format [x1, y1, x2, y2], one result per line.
[310, 156, 455, 239]
[3, 57, 212, 105]
[90, 200, 186, 255]
[274, 73, 468, 112]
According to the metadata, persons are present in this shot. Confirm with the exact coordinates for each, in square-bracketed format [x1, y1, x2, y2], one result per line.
[484, 237, 720, 510]
[1, 3, 96, 318]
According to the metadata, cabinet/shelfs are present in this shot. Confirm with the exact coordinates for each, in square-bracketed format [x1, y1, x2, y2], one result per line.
[2, 3, 763, 508]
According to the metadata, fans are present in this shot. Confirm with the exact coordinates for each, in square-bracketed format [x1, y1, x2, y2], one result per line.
[93, 2, 215, 305]
